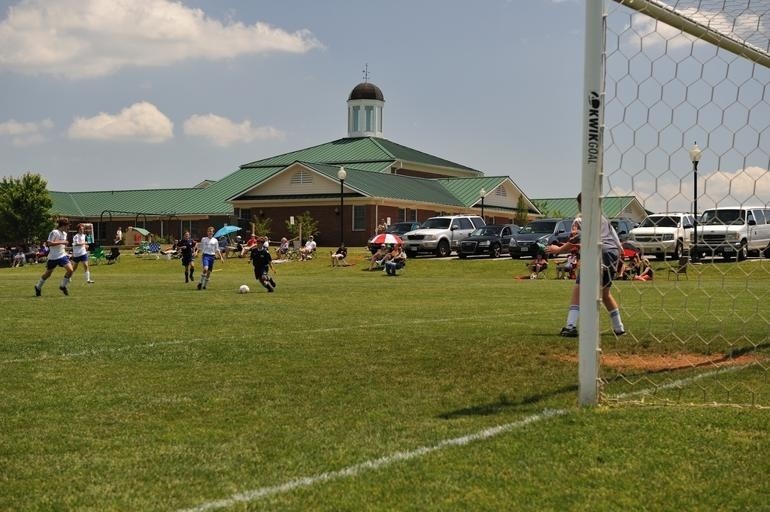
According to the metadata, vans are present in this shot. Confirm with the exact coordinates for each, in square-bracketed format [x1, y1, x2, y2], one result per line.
[690, 205, 770, 260]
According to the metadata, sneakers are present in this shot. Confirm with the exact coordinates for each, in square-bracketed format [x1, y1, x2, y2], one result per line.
[270, 278, 276, 287]
[35, 285, 41, 296]
[561, 324, 578, 337]
[59, 286, 68, 295]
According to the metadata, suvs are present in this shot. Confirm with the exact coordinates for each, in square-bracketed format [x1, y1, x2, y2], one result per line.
[402, 212, 487, 257]
[629, 211, 702, 260]
[508, 216, 574, 260]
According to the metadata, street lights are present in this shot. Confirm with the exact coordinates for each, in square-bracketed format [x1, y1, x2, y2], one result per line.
[337, 164, 349, 250]
[688, 138, 704, 267]
[479, 186, 486, 220]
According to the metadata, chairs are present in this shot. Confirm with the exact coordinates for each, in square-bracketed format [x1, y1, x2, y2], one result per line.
[527, 254, 579, 277]
[274, 240, 317, 262]
[370, 247, 407, 275]
[88, 243, 121, 265]
[135, 240, 162, 264]
[668, 257, 689, 282]
[221, 241, 247, 259]
[336, 247, 348, 267]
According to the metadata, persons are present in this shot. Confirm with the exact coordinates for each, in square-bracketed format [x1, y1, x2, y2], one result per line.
[64, 225, 95, 284]
[248, 238, 277, 293]
[85, 229, 93, 244]
[34, 219, 74, 296]
[379, 219, 387, 231]
[161, 227, 224, 290]
[299, 236, 316, 262]
[12, 241, 50, 268]
[543, 191, 628, 337]
[522, 226, 652, 281]
[114, 226, 123, 244]
[367, 244, 407, 276]
[218, 234, 269, 258]
[275, 237, 289, 260]
[330, 241, 347, 268]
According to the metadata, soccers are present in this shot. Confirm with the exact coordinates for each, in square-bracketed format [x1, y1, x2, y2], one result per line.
[239, 285, 249, 294]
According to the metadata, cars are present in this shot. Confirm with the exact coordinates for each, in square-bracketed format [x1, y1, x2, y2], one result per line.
[369, 220, 422, 257]
[608, 218, 634, 246]
[456, 223, 521, 259]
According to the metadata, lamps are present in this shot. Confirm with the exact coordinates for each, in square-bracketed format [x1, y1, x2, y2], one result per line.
[334, 207, 339, 214]
[259, 208, 264, 216]
